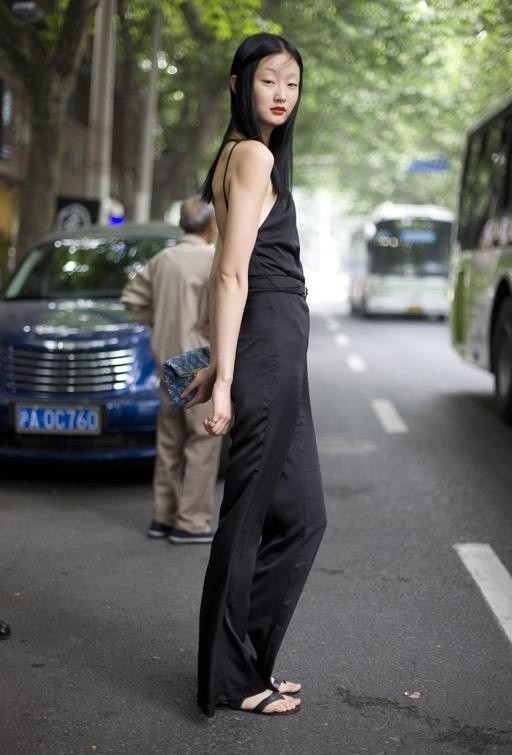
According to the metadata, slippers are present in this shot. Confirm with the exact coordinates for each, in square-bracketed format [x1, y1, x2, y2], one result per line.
[270, 677, 302, 696]
[215, 686, 303, 716]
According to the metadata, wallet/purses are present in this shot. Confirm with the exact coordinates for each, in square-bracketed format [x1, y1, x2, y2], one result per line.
[161, 346, 211, 410]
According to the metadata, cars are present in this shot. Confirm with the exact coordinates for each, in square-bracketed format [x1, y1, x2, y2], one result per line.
[0, 223, 181, 461]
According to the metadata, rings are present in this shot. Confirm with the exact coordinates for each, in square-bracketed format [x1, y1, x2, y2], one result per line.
[208, 417, 218, 425]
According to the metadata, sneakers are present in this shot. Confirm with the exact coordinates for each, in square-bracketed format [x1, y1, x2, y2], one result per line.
[169, 524, 215, 544]
[149, 518, 174, 538]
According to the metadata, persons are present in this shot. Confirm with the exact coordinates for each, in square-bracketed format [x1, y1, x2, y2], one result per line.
[119, 193, 221, 546]
[176, 31, 331, 717]
